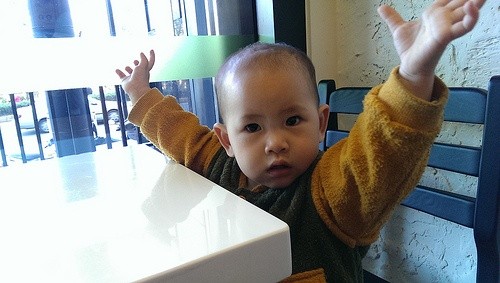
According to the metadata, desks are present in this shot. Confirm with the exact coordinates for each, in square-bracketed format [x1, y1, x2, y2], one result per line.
[0, 145, 293, 283]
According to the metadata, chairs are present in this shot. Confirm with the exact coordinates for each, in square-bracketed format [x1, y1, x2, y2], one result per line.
[306, 72, 499, 283]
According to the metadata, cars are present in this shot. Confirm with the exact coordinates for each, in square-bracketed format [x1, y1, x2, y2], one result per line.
[15, 94, 135, 133]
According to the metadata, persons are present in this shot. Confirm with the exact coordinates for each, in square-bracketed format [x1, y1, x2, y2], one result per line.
[115, 0, 486, 283]
